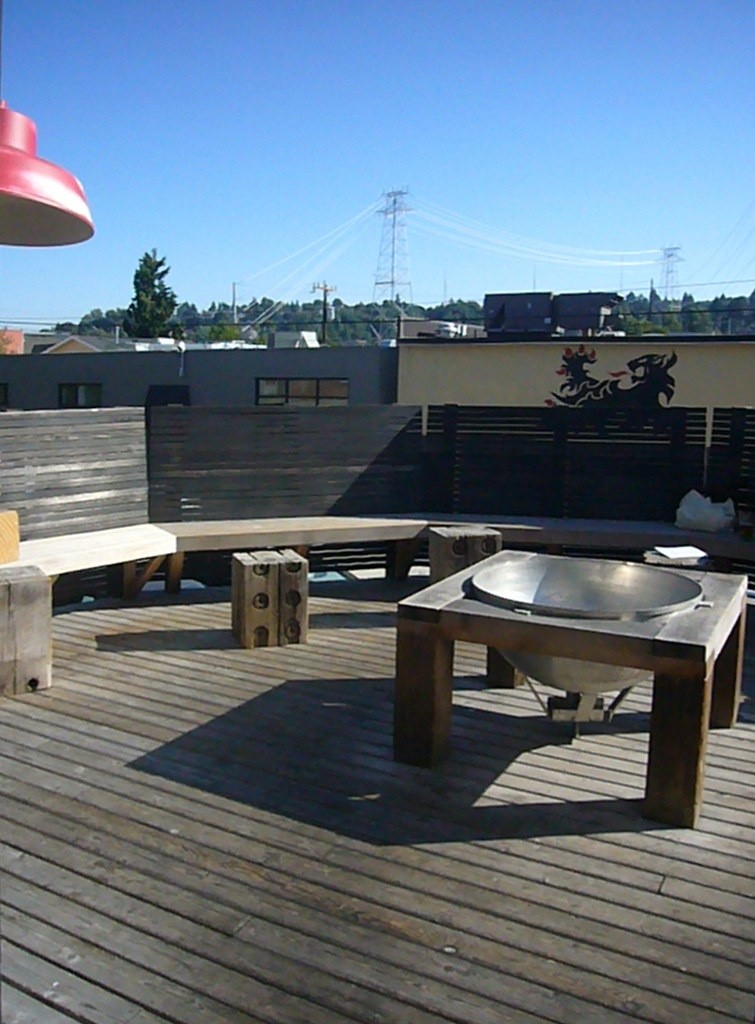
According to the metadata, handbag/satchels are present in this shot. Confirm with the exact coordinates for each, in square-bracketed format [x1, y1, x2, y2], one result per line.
[675, 489, 734, 532]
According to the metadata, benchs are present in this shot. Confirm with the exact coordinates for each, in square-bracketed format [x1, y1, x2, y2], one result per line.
[0, 405, 755, 613]
